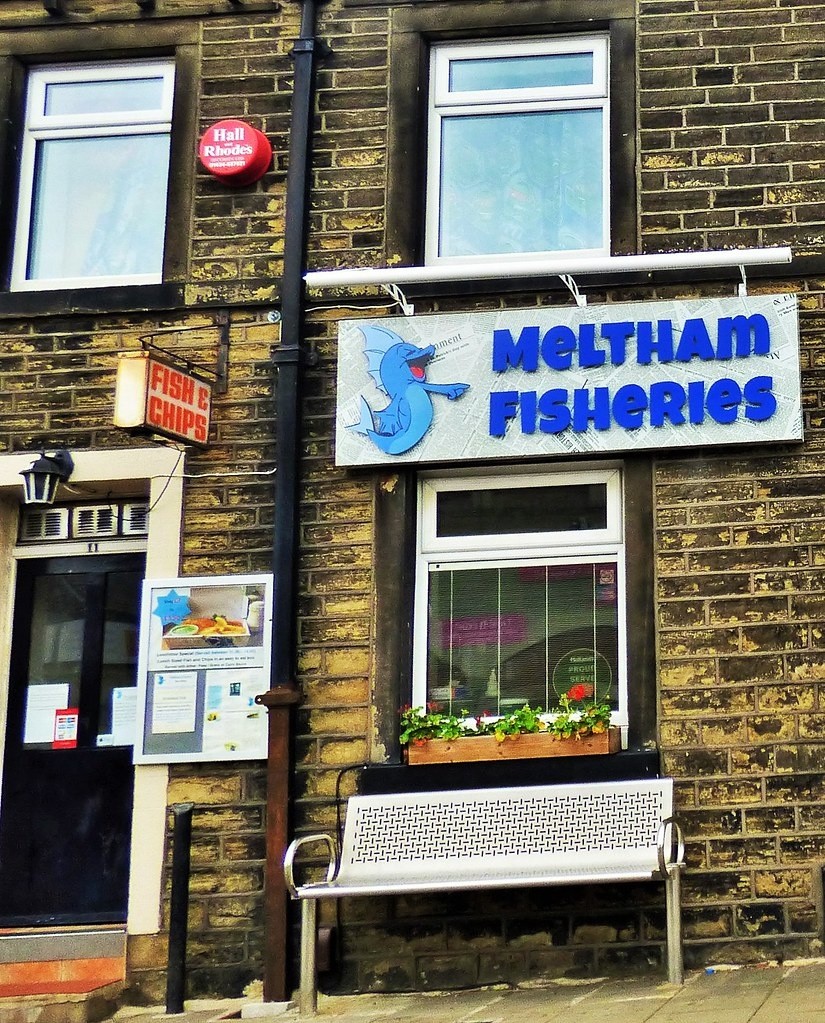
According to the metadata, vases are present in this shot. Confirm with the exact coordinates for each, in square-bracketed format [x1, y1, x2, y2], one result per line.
[407, 726, 621, 765]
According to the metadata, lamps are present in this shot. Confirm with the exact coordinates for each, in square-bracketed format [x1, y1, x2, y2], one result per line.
[19, 443, 68, 506]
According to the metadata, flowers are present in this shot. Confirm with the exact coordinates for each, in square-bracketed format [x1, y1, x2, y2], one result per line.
[397, 685, 611, 741]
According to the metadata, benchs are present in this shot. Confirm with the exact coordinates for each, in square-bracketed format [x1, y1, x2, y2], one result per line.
[283, 777, 685, 1017]
